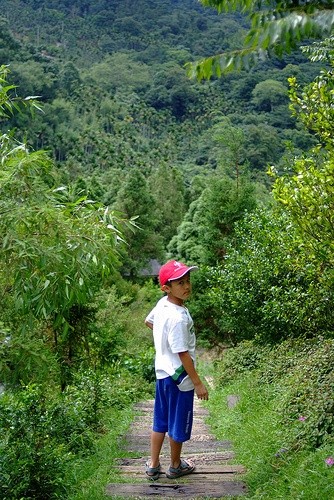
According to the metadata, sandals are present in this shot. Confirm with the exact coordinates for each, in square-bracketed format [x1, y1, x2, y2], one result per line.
[145, 459, 161, 480]
[166, 459, 196, 479]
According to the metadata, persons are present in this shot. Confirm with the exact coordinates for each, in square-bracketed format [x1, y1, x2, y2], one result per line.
[144, 260, 210, 481]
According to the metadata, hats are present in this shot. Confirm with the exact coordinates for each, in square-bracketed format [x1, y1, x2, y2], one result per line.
[159, 260, 198, 286]
